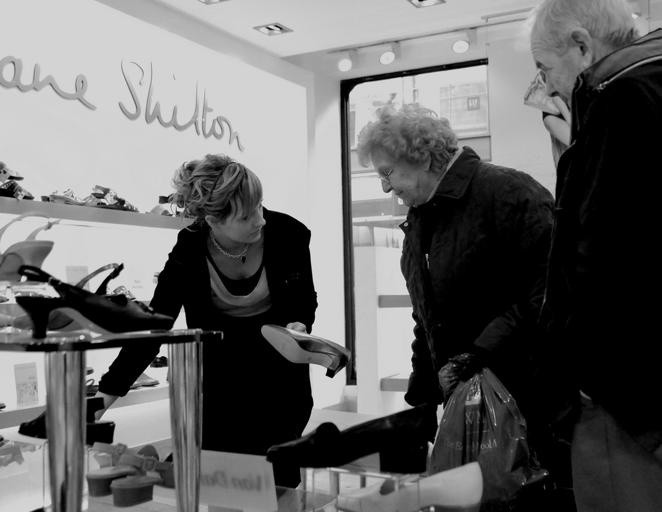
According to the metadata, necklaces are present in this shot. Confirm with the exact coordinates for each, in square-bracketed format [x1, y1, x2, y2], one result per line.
[210, 230, 250, 264]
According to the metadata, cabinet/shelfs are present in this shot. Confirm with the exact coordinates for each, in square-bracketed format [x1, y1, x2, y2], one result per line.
[0, 196, 197, 431]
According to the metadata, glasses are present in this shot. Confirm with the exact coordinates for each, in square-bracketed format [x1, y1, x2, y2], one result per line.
[381, 168, 393, 184]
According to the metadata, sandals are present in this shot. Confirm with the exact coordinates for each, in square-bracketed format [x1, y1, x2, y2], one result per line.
[0, 401, 36, 467]
[0, 293, 15, 329]
[0, 161, 174, 216]
[85, 354, 169, 397]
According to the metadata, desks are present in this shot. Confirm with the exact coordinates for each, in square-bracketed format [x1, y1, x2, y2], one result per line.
[0, 325, 228, 511]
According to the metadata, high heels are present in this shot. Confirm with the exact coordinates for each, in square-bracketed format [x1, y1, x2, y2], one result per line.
[261, 324, 353, 378]
[110, 450, 176, 508]
[335, 460, 483, 512]
[85, 443, 160, 498]
[18, 397, 116, 445]
[14, 262, 175, 343]
[265, 401, 438, 475]
[0, 211, 60, 281]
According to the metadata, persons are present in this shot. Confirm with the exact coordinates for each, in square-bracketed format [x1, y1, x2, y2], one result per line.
[93, 153, 318, 456]
[512, 1, 662, 512]
[355, 103, 582, 512]
